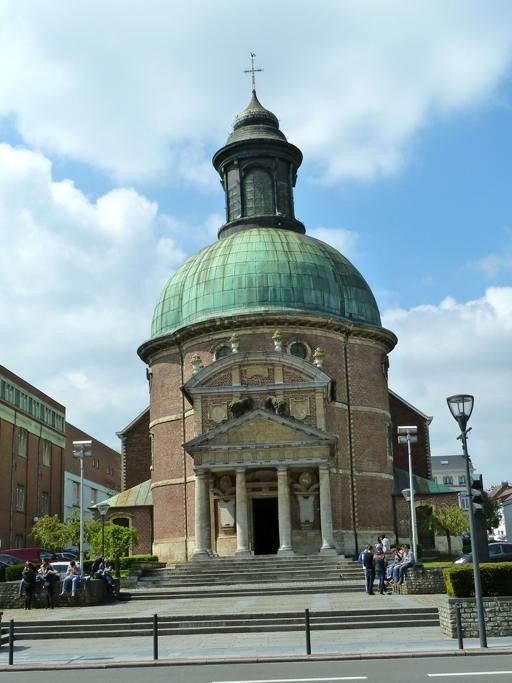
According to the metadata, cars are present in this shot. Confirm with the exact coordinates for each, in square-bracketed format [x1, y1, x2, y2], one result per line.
[449, 541, 512, 565]
[0, 554, 25, 582]
[56, 552, 77, 559]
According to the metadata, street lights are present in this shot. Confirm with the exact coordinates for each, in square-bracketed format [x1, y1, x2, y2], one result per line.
[72, 440, 94, 578]
[96, 502, 111, 571]
[401, 488, 417, 552]
[395, 425, 420, 564]
[446, 394, 491, 647]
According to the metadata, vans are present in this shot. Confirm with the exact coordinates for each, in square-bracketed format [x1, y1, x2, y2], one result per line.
[38, 562, 85, 579]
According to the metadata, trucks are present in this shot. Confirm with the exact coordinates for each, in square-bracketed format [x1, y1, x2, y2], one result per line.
[0, 547, 74, 563]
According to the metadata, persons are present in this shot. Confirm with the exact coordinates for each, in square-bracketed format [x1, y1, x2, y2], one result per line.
[359, 532, 418, 595]
[19, 554, 120, 609]
